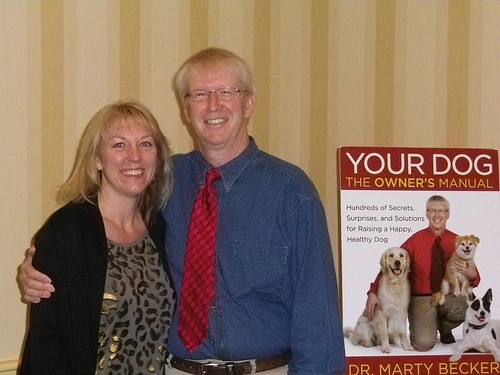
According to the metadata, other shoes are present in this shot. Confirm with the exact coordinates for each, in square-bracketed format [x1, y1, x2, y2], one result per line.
[438, 326, 456, 344]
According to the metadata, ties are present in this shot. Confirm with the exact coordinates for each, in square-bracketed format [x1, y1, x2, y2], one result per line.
[177, 165, 220, 354]
[429, 235, 445, 292]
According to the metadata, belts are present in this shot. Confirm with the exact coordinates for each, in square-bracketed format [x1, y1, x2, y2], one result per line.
[411, 293, 432, 296]
[163, 351, 290, 375]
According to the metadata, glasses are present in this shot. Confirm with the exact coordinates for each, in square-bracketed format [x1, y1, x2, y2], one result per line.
[184, 87, 246, 101]
[426, 208, 448, 214]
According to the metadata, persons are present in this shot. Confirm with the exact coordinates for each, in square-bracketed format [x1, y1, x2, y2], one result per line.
[367, 196, 482, 352]
[17, 102, 178, 375]
[17, 49, 346, 375]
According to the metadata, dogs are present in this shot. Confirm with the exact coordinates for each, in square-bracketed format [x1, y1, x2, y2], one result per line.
[438, 235, 482, 306]
[343, 247, 416, 355]
[449, 288, 500, 365]
